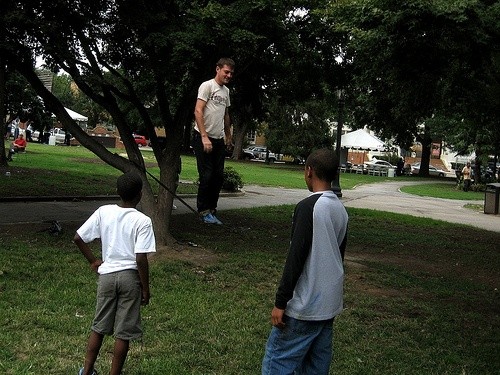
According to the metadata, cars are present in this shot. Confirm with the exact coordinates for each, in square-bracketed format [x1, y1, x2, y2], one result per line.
[225, 145, 254, 161]
[243, 146, 354, 170]
[411, 163, 447, 177]
[363, 160, 398, 172]
[133, 135, 147, 148]
[11, 123, 73, 146]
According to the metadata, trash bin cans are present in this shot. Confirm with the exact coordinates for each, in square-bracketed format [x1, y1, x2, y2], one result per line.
[484, 183, 500, 214]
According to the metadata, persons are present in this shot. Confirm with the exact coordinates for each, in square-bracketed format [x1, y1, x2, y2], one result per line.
[260, 148, 348, 375]
[264, 147, 270, 165]
[73, 173, 156, 375]
[7, 133, 27, 162]
[397, 158, 404, 175]
[13, 119, 71, 146]
[192, 56, 234, 227]
[462, 162, 472, 193]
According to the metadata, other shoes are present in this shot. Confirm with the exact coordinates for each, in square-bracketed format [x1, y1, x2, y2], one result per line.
[79, 367, 97, 375]
[173, 205, 177, 210]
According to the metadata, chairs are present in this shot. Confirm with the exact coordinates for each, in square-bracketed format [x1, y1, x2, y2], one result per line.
[11, 142, 28, 159]
[340, 163, 412, 177]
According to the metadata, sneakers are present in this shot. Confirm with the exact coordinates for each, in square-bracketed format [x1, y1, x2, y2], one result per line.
[211, 210, 224, 224]
[199, 209, 217, 223]
